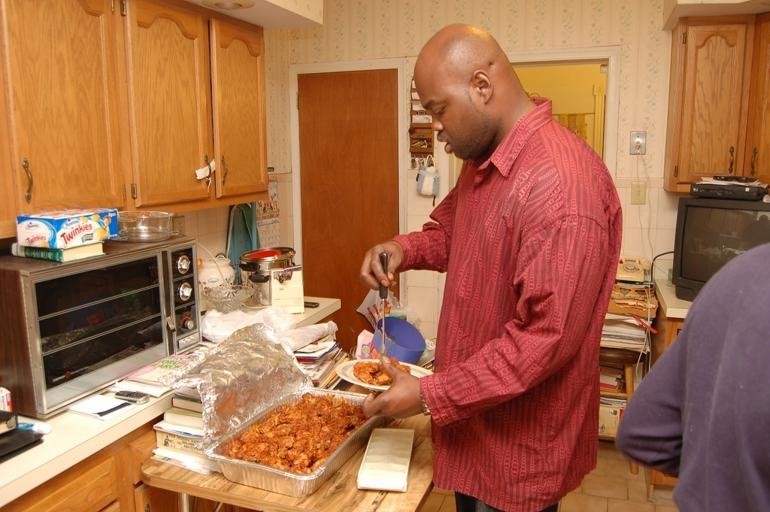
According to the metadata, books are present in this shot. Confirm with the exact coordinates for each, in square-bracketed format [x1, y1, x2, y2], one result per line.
[0, 410, 43, 463]
[597, 312, 652, 407]
[9, 243, 107, 263]
[70, 353, 224, 475]
[293, 335, 350, 391]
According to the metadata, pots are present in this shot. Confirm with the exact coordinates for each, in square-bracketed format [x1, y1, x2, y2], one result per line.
[238, 246, 296, 306]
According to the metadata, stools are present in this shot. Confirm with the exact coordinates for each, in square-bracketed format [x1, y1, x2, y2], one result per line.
[595, 345, 645, 474]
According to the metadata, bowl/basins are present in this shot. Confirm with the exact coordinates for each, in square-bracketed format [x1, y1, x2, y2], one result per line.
[118, 210, 172, 242]
[201, 285, 255, 313]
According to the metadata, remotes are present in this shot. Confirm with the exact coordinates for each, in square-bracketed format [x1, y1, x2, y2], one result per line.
[713, 176, 756, 182]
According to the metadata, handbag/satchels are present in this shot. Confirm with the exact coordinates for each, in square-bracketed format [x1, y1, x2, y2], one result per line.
[417, 155, 439, 196]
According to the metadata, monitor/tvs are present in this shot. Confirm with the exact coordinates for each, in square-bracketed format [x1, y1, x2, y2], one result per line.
[672, 196, 770, 302]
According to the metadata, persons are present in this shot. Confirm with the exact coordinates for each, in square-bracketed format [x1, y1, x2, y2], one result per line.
[360, 23, 622, 512]
[613, 240, 769, 512]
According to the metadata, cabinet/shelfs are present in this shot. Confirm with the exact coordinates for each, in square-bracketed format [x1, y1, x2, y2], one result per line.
[125, 427, 237, 512]
[647, 268, 693, 502]
[0, 449, 125, 511]
[122, 1, 270, 219]
[0, 0, 128, 241]
[662, 10, 770, 195]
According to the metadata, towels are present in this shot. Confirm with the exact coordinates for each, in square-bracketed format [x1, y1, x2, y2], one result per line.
[228, 203, 261, 286]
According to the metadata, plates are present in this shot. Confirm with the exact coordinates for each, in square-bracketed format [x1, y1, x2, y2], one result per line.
[333, 359, 434, 393]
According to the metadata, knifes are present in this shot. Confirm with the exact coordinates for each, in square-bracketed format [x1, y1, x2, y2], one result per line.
[379, 250, 389, 356]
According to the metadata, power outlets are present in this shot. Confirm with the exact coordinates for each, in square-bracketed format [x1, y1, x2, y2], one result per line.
[629, 131, 646, 155]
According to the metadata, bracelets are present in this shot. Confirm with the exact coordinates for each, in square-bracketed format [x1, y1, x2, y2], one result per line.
[420, 393, 431, 415]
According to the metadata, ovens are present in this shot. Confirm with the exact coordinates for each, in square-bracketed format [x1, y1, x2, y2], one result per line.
[0, 234, 205, 419]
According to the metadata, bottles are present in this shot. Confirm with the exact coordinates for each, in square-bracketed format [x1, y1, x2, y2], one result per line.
[200, 258, 235, 284]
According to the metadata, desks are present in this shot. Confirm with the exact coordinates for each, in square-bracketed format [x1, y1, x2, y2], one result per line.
[140, 379, 436, 511]
